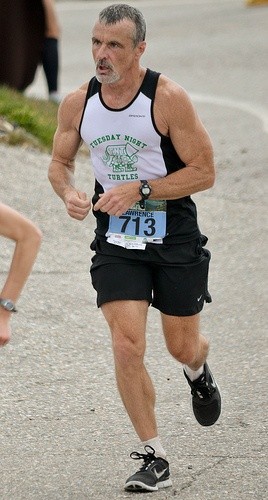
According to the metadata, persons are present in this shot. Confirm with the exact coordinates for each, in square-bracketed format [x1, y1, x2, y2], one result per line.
[48, 4, 221, 491]
[40, 0, 64, 106]
[0, 202, 43, 347]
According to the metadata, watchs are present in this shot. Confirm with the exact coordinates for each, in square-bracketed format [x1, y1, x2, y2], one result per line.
[138, 180, 152, 201]
[0, 298, 19, 313]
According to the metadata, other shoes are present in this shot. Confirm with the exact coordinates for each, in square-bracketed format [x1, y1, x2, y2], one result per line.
[49, 91, 63, 105]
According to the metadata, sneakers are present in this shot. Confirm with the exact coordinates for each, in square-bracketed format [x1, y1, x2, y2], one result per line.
[183, 361, 221, 426]
[124, 445, 172, 491]
